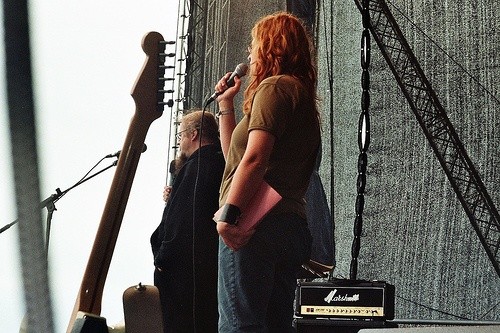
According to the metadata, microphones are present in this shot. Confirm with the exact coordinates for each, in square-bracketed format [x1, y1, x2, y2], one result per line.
[207, 63, 248, 104]
[106, 144, 147, 158]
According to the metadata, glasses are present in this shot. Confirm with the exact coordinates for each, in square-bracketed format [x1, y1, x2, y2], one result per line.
[175, 129, 190, 139]
[248, 46, 255, 53]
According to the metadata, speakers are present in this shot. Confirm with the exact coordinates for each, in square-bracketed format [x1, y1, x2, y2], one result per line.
[70, 311, 109, 333]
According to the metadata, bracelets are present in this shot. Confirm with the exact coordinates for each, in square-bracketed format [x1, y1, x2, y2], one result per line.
[218, 204, 244, 226]
[216, 109, 234, 116]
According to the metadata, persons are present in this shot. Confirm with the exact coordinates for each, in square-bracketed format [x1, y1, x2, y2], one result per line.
[151, 112, 225, 333]
[215, 12, 326, 333]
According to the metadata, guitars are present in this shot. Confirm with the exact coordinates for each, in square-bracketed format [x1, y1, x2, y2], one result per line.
[66, 31, 177, 333]
